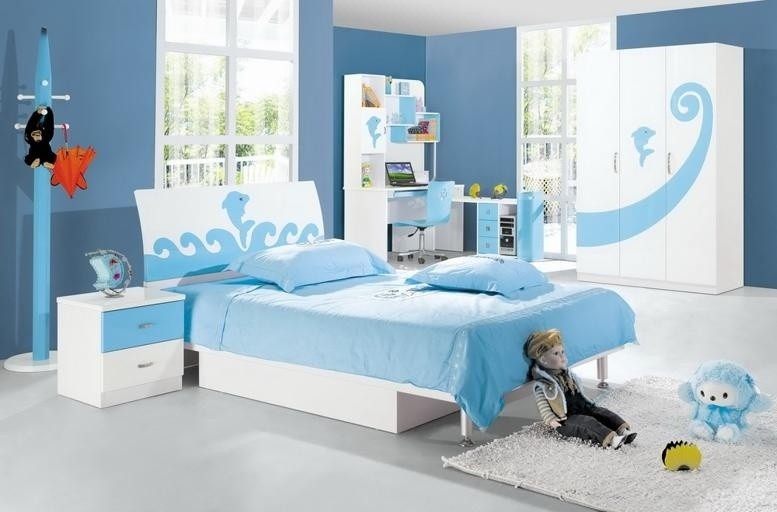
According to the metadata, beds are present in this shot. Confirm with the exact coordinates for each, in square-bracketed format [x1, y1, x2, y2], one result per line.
[132, 181, 638, 449]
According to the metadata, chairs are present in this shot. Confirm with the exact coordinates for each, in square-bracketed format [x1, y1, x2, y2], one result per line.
[387, 181, 455, 263]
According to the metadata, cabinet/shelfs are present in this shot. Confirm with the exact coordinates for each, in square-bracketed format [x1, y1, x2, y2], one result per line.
[573, 43, 745, 295]
[342, 73, 428, 188]
[476, 191, 544, 262]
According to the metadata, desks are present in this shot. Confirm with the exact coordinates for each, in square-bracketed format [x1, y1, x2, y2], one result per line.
[347, 182, 465, 261]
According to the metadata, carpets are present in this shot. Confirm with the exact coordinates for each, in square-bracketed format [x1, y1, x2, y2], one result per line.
[436, 373, 776, 511]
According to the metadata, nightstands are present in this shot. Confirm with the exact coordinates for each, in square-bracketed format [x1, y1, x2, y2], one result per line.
[56, 286, 186, 408]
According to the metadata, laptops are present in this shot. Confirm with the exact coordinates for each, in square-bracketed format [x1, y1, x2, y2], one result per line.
[385, 162, 428, 186]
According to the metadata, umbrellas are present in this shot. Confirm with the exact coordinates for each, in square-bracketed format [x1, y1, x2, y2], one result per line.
[46, 123, 97, 200]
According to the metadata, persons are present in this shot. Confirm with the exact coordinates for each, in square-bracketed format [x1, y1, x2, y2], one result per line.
[522, 327, 637, 449]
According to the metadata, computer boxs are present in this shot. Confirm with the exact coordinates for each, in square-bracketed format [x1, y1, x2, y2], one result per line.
[500, 215, 516, 257]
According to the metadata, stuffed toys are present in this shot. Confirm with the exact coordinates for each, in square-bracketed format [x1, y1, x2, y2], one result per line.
[660, 440, 703, 472]
[22, 105, 56, 170]
[675, 358, 774, 445]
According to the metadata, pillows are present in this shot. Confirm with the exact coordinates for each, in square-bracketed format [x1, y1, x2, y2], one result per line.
[404, 252, 546, 294]
[217, 236, 396, 293]
